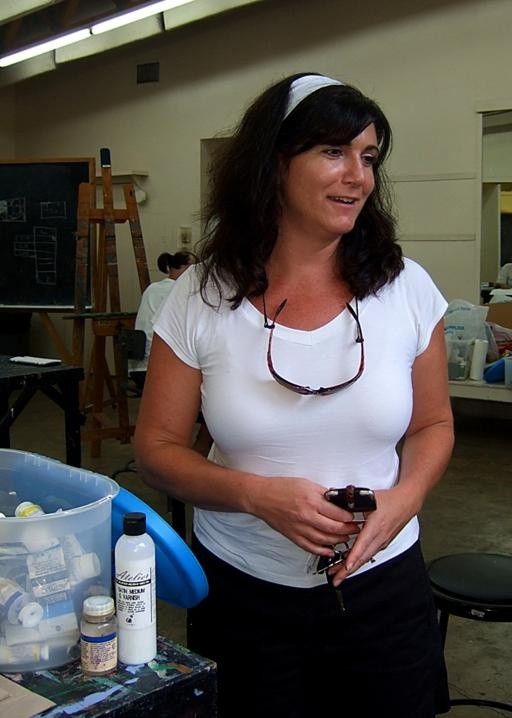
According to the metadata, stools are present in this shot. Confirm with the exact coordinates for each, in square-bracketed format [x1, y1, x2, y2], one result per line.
[424, 549, 511, 711]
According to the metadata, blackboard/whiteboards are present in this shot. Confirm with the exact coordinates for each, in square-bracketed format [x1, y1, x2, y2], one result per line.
[1, 157, 94, 311]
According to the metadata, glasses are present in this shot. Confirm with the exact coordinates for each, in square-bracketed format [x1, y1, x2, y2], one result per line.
[267, 296, 365, 397]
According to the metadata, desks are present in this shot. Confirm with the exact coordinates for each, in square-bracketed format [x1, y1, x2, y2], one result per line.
[0, 354, 85, 468]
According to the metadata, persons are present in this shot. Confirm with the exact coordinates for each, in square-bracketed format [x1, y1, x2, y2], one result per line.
[128, 251, 214, 459]
[131, 72, 456, 718]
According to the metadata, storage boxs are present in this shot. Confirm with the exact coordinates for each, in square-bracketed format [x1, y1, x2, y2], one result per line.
[1, 449, 121, 676]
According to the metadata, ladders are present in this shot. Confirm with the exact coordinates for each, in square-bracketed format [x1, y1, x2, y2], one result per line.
[63, 182, 151, 454]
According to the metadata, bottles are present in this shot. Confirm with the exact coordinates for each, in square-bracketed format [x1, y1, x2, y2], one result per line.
[0, 576, 43, 629]
[80, 511, 158, 675]
[14, 501, 45, 516]
